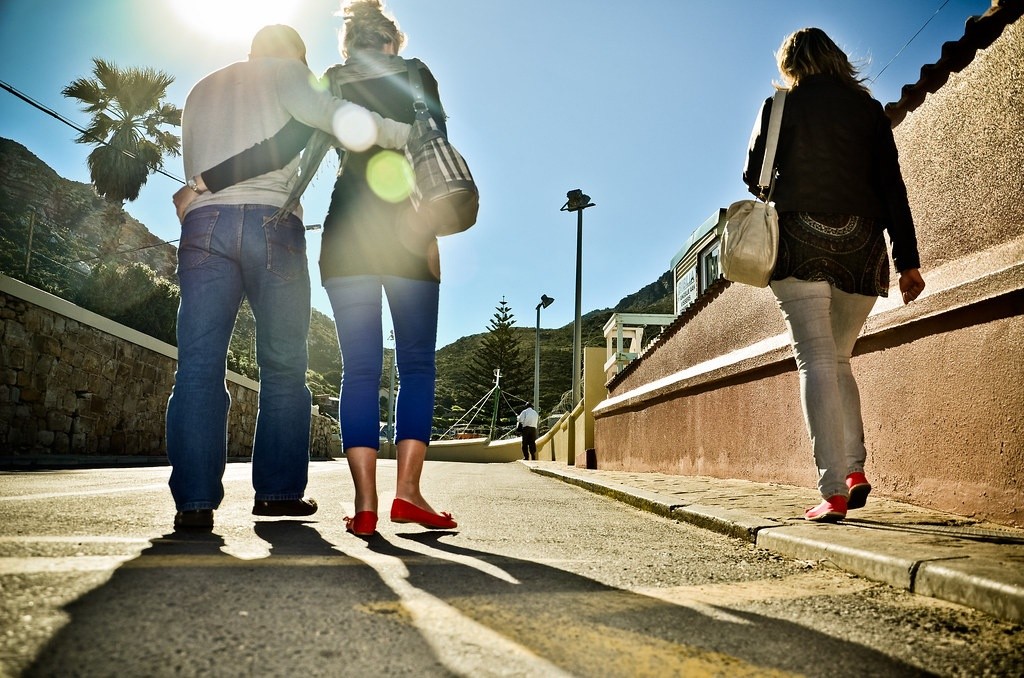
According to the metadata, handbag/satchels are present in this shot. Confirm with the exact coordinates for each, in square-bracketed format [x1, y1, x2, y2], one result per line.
[409, 58, 479, 236]
[717, 200, 780, 288]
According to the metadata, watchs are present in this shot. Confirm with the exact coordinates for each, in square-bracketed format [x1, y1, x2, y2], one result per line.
[188, 176, 202, 194]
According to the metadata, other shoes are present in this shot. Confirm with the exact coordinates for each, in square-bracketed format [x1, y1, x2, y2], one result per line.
[251, 497, 318, 516]
[523, 458, 529, 460]
[174, 508, 215, 529]
[805, 496, 847, 521]
[343, 511, 378, 535]
[531, 456, 535, 460]
[845, 472, 872, 510]
[392, 499, 458, 529]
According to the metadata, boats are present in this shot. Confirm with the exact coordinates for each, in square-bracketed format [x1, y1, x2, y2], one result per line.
[332, 369, 522, 462]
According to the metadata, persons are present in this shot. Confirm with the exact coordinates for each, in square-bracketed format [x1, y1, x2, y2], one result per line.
[173, 0, 458, 535]
[166, 25, 411, 534]
[742, 28, 926, 519]
[517, 402, 539, 460]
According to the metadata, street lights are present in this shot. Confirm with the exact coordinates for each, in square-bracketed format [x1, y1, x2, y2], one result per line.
[559, 189, 596, 410]
[534, 294, 555, 439]
